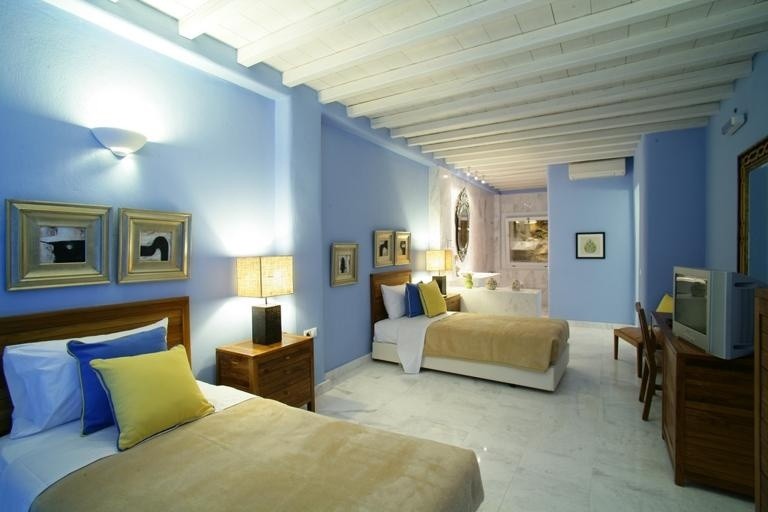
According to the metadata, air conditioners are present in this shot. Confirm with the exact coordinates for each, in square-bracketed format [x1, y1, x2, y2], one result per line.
[568, 158, 626, 180]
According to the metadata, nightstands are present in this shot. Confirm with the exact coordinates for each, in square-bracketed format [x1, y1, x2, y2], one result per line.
[444, 294, 460, 312]
[216, 333, 315, 412]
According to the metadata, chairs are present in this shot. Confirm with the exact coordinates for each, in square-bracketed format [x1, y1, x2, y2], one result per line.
[614, 327, 664, 378]
[635, 301, 663, 422]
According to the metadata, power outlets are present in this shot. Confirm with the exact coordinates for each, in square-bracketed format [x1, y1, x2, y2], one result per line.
[304, 328, 318, 337]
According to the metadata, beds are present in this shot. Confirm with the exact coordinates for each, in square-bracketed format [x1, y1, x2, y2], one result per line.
[0, 297, 484, 512]
[370, 269, 569, 392]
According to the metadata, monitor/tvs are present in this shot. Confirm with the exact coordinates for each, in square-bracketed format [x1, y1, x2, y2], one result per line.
[672, 264, 765, 360]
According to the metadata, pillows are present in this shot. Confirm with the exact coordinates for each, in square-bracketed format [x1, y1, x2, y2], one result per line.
[3, 316, 168, 439]
[647, 293, 673, 333]
[417, 278, 447, 317]
[89, 344, 215, 452]
[380, 281, 409, 320]
[66, 327, 168, 437]
[404, 280, 424, 318]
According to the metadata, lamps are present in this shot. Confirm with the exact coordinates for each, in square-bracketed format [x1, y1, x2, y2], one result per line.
[236, 255, 294, 345]
[90, 126, 148, 157]
[426, 250, 452, 298]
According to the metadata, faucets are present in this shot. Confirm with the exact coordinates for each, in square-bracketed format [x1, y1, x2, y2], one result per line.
[454, 264, 460, 277]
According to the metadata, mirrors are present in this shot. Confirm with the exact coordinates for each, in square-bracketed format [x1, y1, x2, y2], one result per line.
[505, 217, 549, 267]
[456, 187, 469, 262]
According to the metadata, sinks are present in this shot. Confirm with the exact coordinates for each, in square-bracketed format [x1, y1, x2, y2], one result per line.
[463, 271, 500, 287]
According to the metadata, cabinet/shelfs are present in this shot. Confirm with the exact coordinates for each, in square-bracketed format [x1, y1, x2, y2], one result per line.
[650, 310, 754, 502]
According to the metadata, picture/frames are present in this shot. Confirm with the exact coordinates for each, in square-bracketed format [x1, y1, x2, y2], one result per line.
[374, 229, 394, 268]
[5, 198, 112, 292]
[117, 208, 192, 285]
[395, 231, 411, 265]
[330, 242, 359, 287]
[576, 232, 605, 259]
